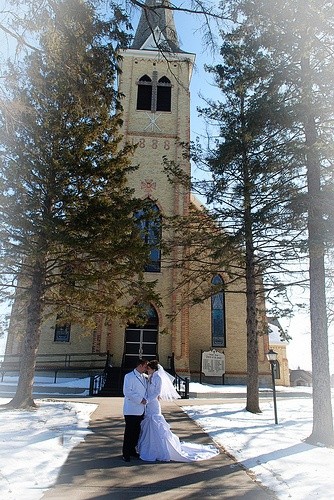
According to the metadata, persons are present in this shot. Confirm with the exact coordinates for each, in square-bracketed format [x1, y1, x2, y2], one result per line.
[122, 359, 161, 462]
[135, 359, 163, 462]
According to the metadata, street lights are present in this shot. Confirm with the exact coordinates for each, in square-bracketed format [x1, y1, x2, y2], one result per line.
[265, 349, 280, 425]
